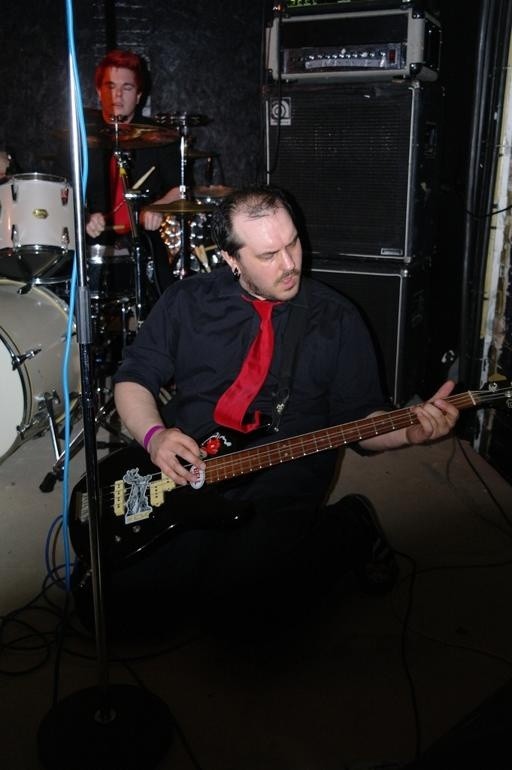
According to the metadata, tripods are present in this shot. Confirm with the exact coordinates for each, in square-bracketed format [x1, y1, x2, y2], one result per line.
[38, 137, 134, 492]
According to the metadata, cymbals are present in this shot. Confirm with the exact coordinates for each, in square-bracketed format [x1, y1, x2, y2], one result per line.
[85, 123, 221, 158]
[142, 186, 232, 216]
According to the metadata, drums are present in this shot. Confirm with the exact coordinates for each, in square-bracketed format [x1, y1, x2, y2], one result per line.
[159, 202, 219, 278]
[84, 243, 153, 300]
[0, 170, 77, 279]
[0, 280, 81, 464]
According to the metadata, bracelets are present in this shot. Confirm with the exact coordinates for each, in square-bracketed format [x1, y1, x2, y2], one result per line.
[144, 420, 165, 451]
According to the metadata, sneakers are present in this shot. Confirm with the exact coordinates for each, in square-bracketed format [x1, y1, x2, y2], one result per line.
[332, 493, 402, 585]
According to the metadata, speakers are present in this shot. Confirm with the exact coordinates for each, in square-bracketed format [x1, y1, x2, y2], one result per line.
[264, 81, 440, 254]
[304, 255, 431, 408]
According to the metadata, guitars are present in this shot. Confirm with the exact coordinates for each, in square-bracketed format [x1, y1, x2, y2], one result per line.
[68, 379, 512, 567]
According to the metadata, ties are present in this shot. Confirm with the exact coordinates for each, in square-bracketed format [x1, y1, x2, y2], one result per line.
[210, 296, 285, 435]
[108, 153, 132, 235]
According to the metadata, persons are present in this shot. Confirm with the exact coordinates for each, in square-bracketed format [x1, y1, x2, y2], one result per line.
[0, 149, 16, 179]
[80, 48, 190, 292]
[73, 183, 460, 660]
